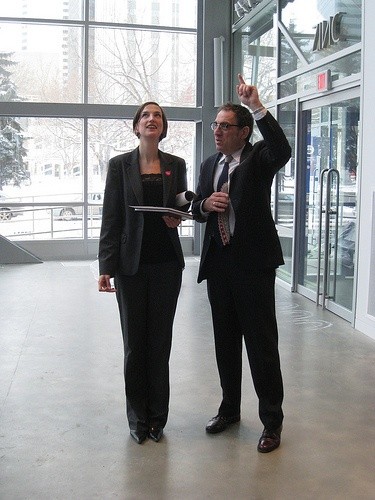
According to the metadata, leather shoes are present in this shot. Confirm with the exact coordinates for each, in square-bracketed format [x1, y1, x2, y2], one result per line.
[257, 423, 283, 453]
[130, 430, 148, 444]
[148, 427, 163, 442]
[206, 414, 240, 434]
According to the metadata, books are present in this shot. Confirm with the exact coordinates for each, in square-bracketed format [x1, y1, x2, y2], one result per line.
[129, 206, 194, 220]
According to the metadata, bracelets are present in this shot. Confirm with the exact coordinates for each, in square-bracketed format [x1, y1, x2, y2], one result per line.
[254, 106, 264, 115]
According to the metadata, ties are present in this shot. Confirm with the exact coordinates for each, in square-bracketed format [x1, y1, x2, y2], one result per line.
[216, 156, 233, 246]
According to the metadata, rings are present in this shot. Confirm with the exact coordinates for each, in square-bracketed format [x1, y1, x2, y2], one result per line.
[213, 202, 216, 206]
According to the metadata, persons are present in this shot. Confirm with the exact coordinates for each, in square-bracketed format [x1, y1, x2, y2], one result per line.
[192, 74, 292, 453]
[98, 102, 191, 445]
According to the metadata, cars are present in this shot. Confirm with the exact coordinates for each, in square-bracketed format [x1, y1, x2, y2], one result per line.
[0, 190, 25, 222]
[271, 192, 317, 224]
[46, 190, 103, 221]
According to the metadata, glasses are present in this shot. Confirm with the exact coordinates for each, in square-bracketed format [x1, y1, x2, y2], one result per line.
[210, 121, 250, 130]
